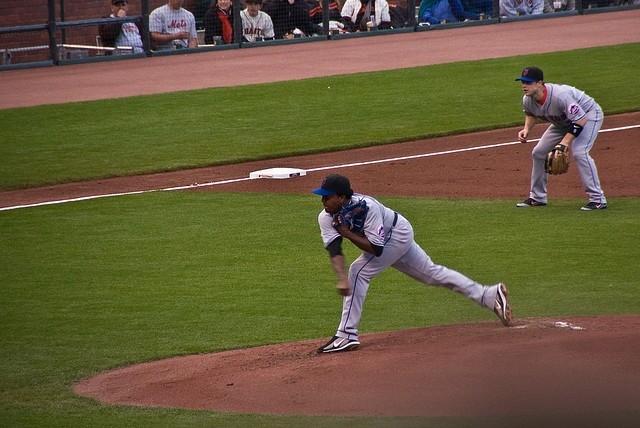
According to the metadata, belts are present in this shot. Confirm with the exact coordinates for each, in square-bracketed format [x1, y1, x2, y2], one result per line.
[393, 213, 398, 227]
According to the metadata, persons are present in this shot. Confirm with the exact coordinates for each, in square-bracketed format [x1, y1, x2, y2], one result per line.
[310, 173, 512, 354]
[239, 0, 276, 42]
[203, 0, 234, 44]
[513, 66, 607, 211]
[149, 0, 199, 50]
[98, 0, 148, 56]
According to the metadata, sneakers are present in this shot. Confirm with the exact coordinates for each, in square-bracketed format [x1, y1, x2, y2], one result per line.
[580, 202, 606, 211]
[494, 283, 513, 327]
[516, 198, 546, 207]
[317, 336, 361, 353]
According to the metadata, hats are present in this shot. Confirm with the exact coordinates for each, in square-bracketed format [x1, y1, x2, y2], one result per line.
[312, 174, 351, 196]
[515, 66, 543, 83]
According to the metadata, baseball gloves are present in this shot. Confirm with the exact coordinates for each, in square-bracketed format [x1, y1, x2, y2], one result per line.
[545, 144, 570, 176]
